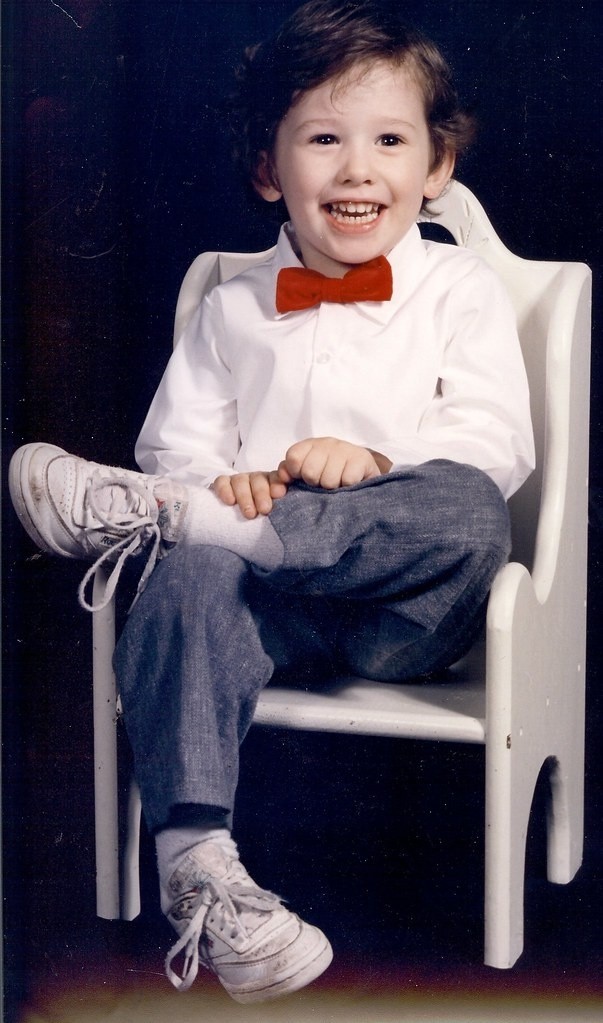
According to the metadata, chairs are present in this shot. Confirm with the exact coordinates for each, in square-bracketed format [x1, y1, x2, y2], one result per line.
[90, 179, 594, 970]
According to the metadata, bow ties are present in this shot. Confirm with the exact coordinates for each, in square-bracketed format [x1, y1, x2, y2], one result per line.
[276, 255, 394, 313]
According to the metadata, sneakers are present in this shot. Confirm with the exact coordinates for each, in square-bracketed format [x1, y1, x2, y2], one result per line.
[165, 838, 334, 1003]
[9, 443, 188, 614]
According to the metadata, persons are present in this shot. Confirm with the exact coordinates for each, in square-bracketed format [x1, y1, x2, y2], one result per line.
[8, 0, 539, 1008]
